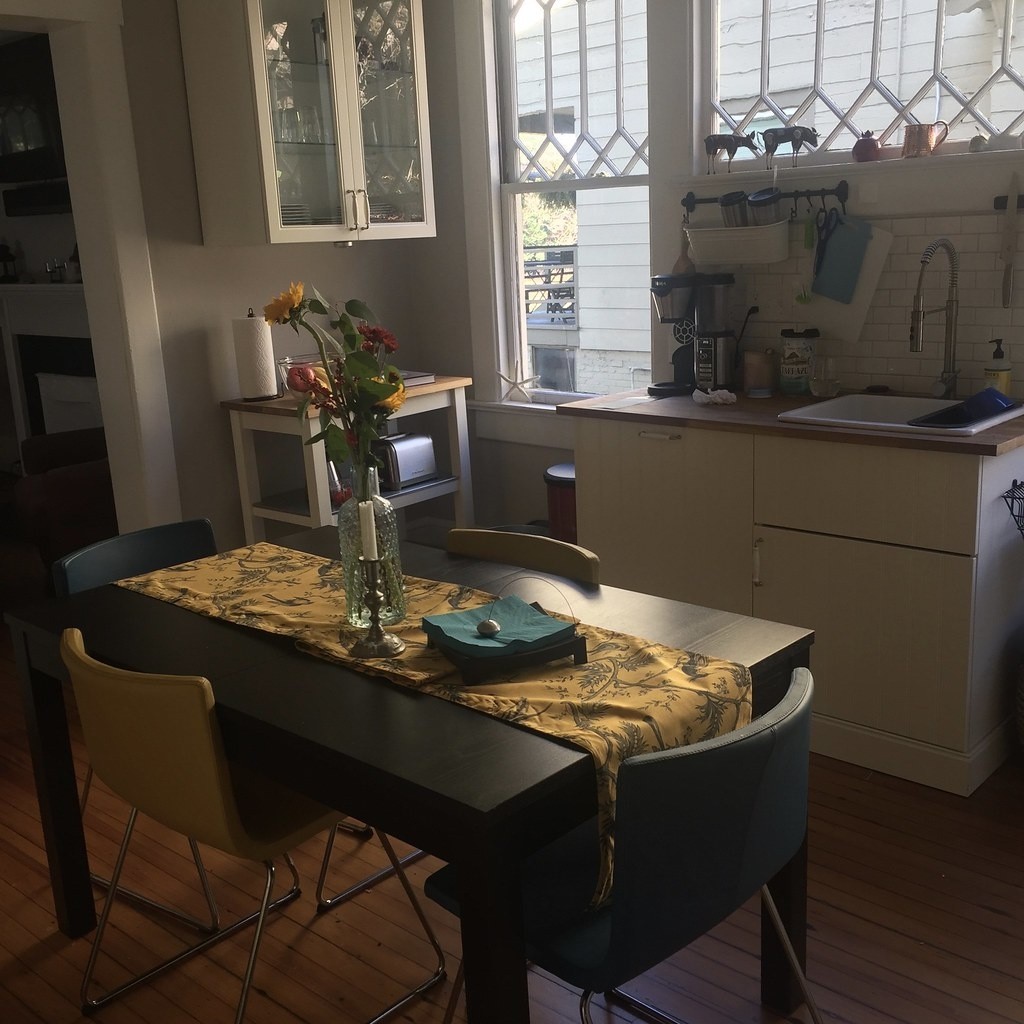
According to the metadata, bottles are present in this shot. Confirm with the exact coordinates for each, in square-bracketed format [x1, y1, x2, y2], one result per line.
[743, 336, 776, 399]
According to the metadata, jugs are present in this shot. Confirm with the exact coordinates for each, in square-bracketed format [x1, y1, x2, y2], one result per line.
[902, 121, 949, 157]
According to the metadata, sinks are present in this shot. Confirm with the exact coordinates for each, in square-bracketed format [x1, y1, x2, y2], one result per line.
[777, 393, 1024, 436]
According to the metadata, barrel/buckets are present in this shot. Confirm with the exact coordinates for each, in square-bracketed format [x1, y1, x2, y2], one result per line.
[779, 329, 821, 395]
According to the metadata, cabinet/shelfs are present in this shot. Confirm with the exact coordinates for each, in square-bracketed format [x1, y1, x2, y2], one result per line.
[572, 421, 754, 613]
[756, 432, 982, 750]
[177, 0, 433, 245]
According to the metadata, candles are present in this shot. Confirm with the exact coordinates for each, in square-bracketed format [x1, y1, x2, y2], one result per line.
[359, 498, 378, 558]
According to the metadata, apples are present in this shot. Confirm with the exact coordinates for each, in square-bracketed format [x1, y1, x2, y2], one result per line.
[851, 130, 881, 162]
[288, 366, 315, 391]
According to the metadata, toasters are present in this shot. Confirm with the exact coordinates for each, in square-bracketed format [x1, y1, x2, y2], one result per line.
[369, 432, 438, 491]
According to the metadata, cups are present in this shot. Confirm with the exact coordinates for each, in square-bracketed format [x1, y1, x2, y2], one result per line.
[274, 105, 411, 144]
[808, 355, 843, 397]
[748, 186, 781, 226]
[718, 191, 747, 227]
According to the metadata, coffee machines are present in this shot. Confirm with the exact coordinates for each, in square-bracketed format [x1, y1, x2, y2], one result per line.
[649, 273, 734, 397]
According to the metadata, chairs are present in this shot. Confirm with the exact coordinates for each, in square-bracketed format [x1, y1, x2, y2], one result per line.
[425, 668, 828, 1024]
[50, 518, 301, 1014]
[58, 620, 451, 1023]
[313, 529, 601, 912]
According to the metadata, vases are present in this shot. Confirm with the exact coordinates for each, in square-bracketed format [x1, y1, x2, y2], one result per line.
[327, 491, 400, 631]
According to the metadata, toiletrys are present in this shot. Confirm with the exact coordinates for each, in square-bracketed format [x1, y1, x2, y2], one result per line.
[983, 338, 1012, 399]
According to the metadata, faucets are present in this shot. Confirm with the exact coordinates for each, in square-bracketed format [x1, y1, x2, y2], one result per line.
[909, 237, 962, 400]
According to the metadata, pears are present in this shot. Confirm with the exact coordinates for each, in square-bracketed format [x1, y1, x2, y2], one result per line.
[968, 125, 990, 152]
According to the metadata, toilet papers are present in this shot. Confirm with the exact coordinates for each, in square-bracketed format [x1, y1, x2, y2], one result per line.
[231, 315, 278, 399]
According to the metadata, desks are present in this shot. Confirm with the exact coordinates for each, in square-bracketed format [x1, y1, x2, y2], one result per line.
[216, 372, 478, 569]
[6, 533, 814, 1024]
[0, 281, 97, 477]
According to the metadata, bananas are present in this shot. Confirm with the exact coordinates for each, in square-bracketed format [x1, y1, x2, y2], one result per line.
[311, 366, 335, 394]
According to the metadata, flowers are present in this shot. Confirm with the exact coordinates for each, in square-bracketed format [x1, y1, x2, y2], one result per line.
[259, 277, 406, 500]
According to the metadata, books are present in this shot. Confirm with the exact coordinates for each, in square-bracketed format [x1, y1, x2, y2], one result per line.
[396, 369, 435, 387]
